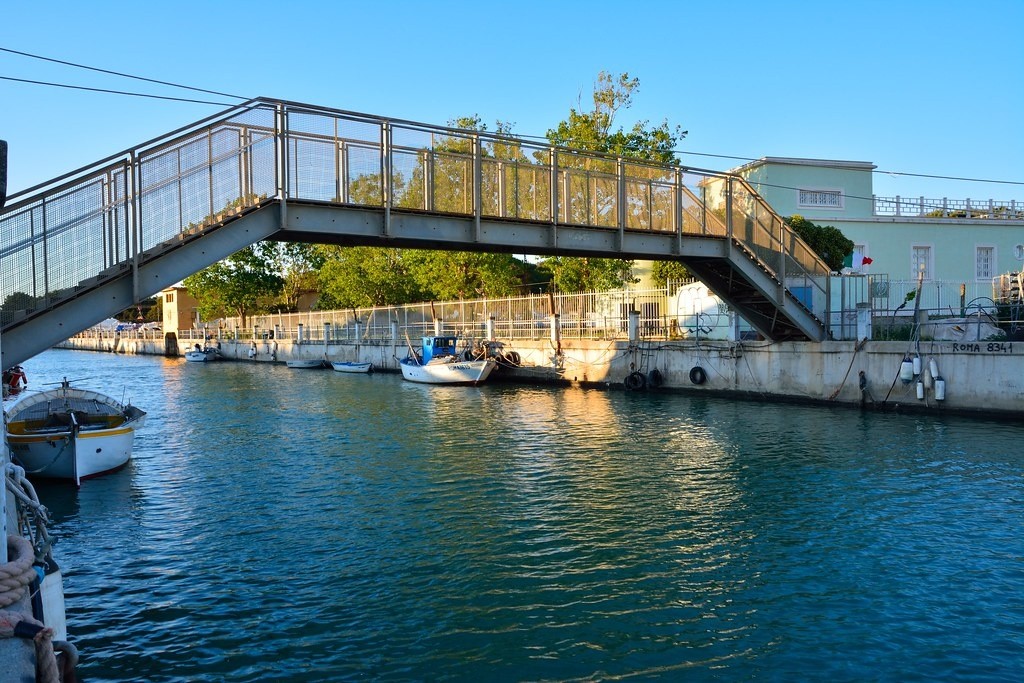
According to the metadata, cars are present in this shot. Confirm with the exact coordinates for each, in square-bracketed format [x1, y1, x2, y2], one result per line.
[264, 330, 284, 339]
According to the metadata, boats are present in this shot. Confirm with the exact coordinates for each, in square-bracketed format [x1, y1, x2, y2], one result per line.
[3, 376, 147, 486]
[331, 361, 372, 372]
[184, 349, 216, 362]
[286, 358, 325, 368]
[400, 336, 497, 384]
